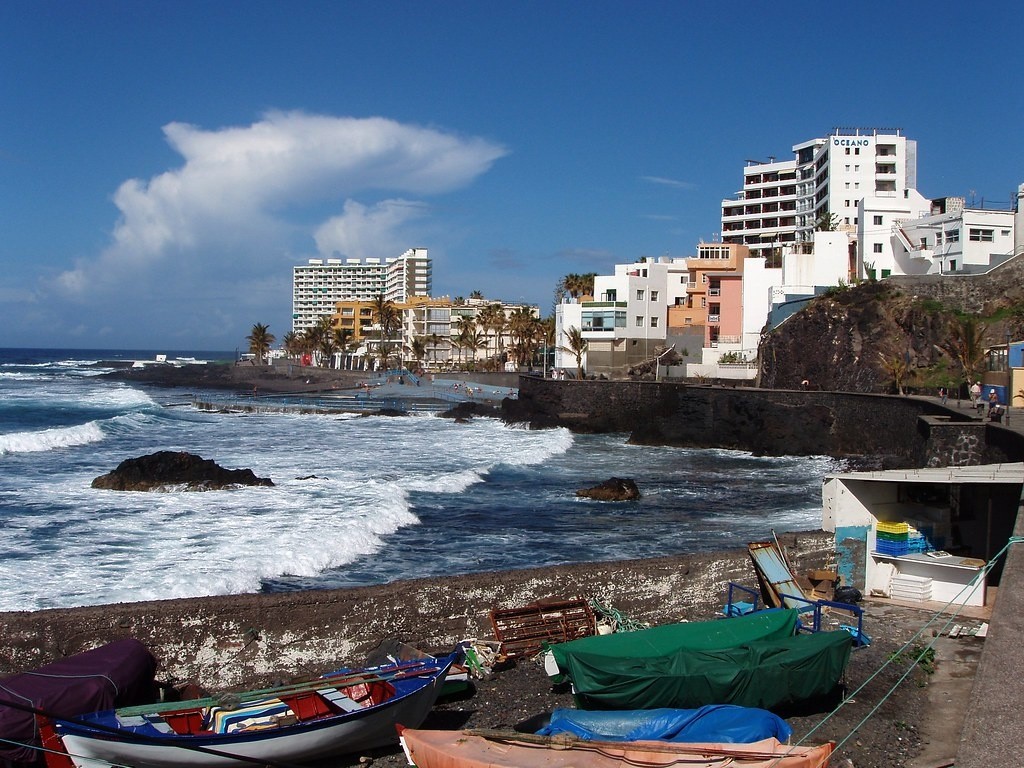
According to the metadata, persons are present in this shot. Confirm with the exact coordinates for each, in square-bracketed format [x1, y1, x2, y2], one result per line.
[453, 381, 518, 399]
[551, 366, 565, 381]
[360, 378, 391, 399]
[971, 381, 980, 409]
[986, 388, 998, 418]
[939, 383, 949, 405]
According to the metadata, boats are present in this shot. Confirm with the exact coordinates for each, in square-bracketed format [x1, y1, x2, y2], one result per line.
[530, 608, 851, 707]
[395, 724, 836, 768]
[44, 645, 461, 768]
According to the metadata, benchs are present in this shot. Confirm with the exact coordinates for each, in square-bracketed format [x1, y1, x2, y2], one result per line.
[991, 408, 1005, 422]
[316, 685, 364, 712]
[145, 712, 175, 734]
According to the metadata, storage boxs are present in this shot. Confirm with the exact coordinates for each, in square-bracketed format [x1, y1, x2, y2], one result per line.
[875, 505, 952, 556]
[891, 574, 932, 604]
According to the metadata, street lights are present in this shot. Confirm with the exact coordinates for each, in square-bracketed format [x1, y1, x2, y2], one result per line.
[542, 332, 546, 380]
